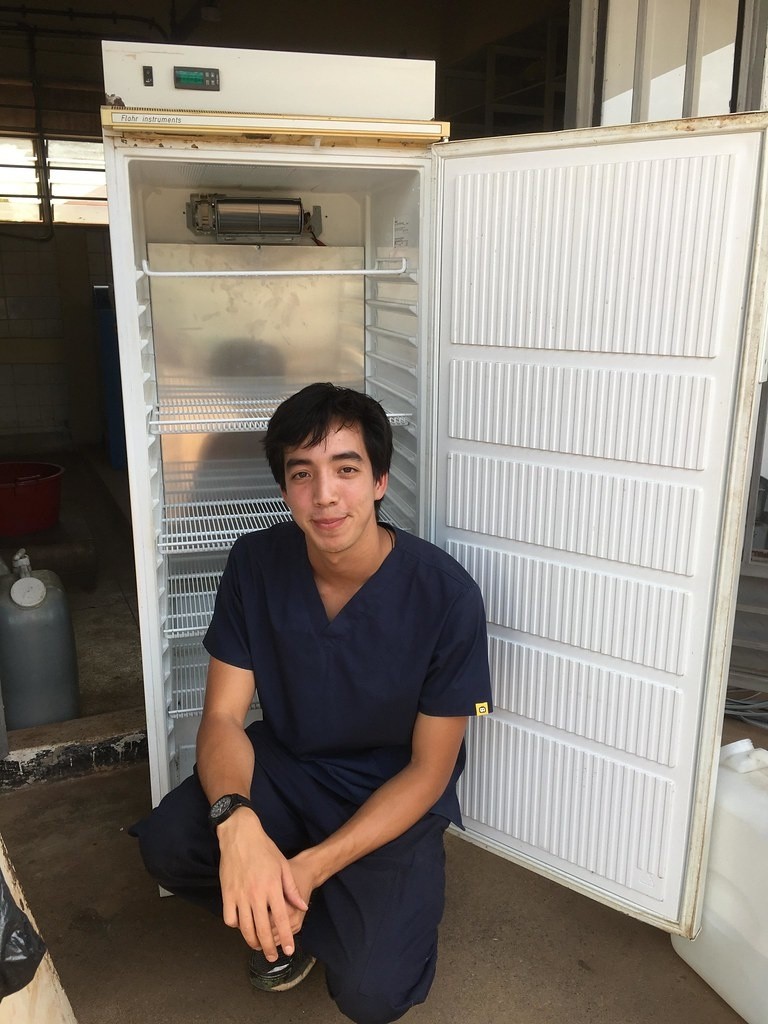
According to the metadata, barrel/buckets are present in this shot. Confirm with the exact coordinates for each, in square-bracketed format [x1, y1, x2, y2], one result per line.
[0, 548, 81, 732]
[670, 737, 768, 1024]
[0, 462, 64, 535]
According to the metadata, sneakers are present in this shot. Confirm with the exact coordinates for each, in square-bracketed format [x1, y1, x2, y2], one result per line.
[245, 938, 319, 992]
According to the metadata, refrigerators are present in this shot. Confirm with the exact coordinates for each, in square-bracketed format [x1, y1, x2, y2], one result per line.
[98, 39, 768, 928]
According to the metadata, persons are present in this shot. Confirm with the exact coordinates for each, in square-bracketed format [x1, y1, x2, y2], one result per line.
[129, 383, 496, 1024]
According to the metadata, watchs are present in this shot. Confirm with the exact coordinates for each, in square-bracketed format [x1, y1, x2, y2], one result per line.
[209, 793, 254, 830]
